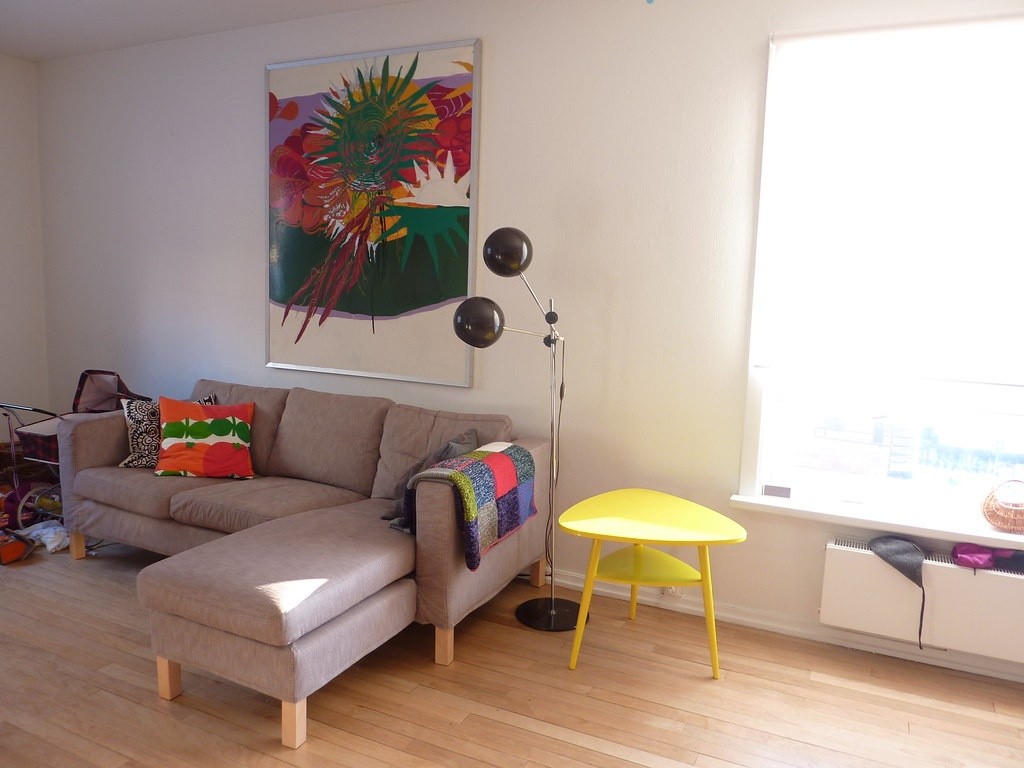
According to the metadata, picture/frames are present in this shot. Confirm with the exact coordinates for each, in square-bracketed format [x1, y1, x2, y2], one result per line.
[263, 37, 482, 389]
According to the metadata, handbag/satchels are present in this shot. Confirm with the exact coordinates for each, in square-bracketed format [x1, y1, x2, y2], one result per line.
[0, 528, 34, 566]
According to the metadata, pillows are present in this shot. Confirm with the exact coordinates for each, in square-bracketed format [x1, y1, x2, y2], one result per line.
[154, 396, 255, 480]
[120, 394, 216, 469]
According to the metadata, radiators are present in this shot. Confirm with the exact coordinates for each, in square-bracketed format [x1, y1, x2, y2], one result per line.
[819, 538, 1024, 664]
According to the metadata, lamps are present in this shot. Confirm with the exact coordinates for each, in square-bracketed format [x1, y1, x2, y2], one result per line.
[454, 228, 591, 631]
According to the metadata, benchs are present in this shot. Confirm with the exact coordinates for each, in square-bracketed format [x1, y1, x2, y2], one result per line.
[58, 377, 552, 749]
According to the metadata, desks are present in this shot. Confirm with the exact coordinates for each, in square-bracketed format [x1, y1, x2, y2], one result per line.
[558, 488, 747, 679]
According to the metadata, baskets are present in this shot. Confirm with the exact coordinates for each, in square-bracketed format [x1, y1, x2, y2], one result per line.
[982, 479, 1024, 535]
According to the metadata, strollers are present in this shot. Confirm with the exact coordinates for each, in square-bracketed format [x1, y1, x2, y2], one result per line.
[0, 369, 153, 549]
